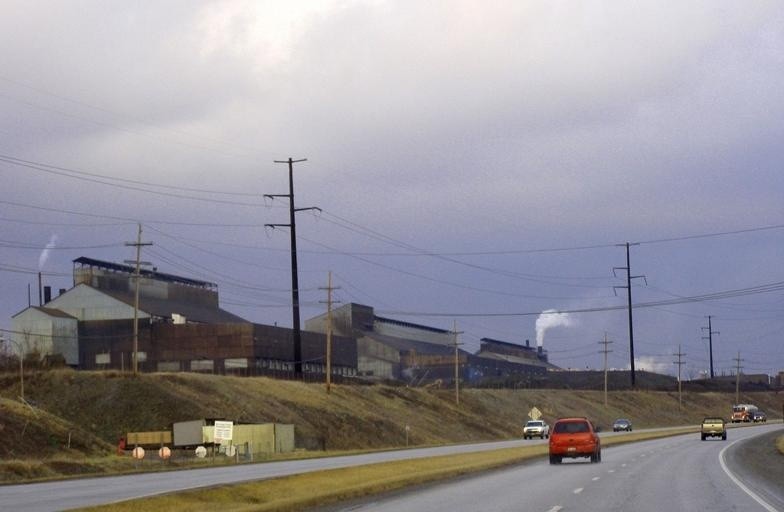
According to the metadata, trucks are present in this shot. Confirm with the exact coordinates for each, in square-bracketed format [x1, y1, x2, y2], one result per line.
[121, 430, 172, 449]
[731, 404, 758, 422]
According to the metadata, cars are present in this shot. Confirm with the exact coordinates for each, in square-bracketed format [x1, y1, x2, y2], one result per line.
[548, 417, 601, 464]
[614, 418, 632, 432]
[753, 412, 766, 422]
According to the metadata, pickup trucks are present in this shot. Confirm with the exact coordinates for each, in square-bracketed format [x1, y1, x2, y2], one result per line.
[701, 418, 726, 440]
[523, 420, 549, 439]
